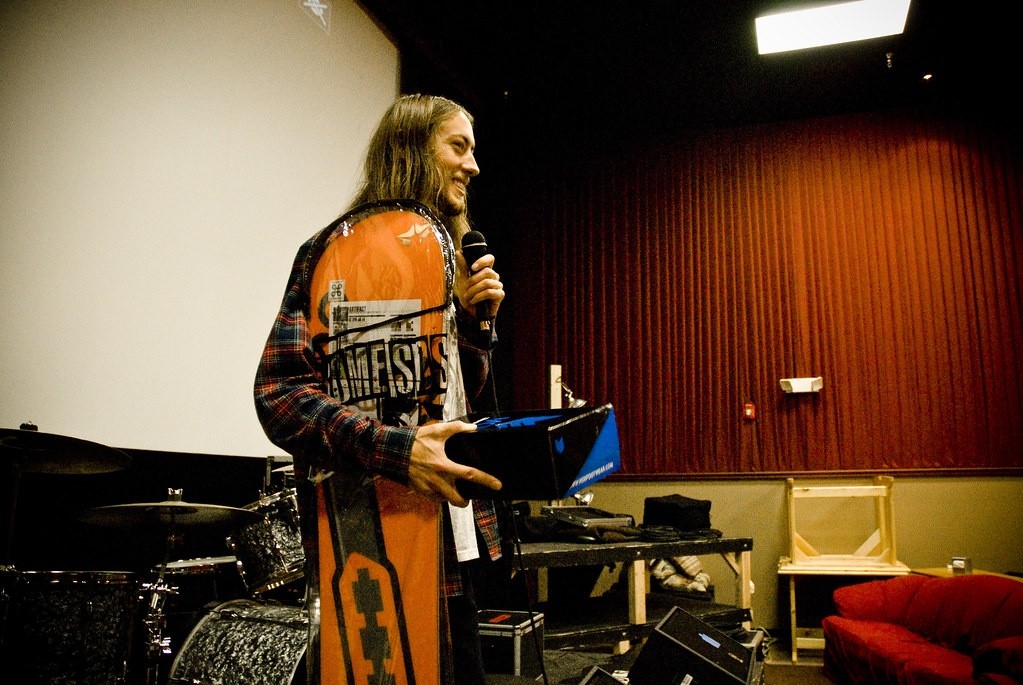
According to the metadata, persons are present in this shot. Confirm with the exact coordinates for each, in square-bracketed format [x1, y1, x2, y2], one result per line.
[254, 94, 505, 685]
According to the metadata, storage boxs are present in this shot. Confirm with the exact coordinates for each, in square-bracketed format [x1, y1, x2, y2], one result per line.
[477, 609, 545, 681]
[446, 404, 622, 501]
[628, 607, 755, 685]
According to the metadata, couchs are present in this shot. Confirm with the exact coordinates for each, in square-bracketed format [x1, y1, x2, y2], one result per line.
[822, 574, 1023, 685]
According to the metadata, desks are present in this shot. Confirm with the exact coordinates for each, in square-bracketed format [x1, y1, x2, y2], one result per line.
[911, 565, 1023, 584]
[482, 538, 753, 648]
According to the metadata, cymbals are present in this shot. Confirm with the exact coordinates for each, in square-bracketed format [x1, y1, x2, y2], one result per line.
[79, 500, 265, 533]
[0, 427, 134, 476]
[271, 463, 294, 473]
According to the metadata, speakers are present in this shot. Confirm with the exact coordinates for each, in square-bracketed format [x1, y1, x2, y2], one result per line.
[574, 604, 752, 685]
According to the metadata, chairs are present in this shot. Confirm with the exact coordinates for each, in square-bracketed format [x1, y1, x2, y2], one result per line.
[777, 476, 911, 663]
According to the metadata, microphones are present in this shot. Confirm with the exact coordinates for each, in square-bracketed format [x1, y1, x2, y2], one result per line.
[462, 230, 496, 346]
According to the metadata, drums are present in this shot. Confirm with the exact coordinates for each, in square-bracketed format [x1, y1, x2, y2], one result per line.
[1, 569, 140, 685]
[154, 554, 240, 617]
[168, 598, 323, 685]
[227, 486, 312, 596]
